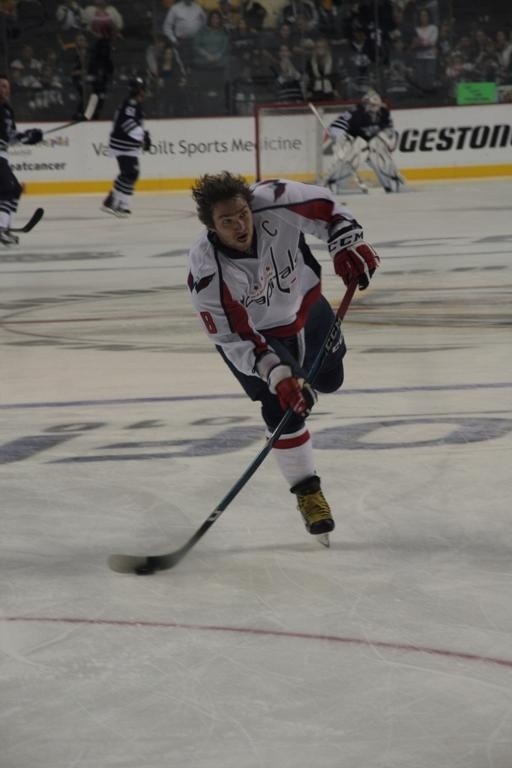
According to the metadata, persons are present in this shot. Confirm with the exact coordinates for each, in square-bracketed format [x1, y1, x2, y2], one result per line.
[323, 85, 401, 193]
[185, 171, 382, 537]
[1, 80, 42, 245]
[101, 76, 152, 219]
[0, 0, 512, 119]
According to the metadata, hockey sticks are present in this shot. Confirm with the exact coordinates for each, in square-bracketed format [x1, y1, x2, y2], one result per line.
[7, 207, 44, 233]
[106, 283, 357, 573]
[308, 102, 369, 194]
[7, 93, 99, 145]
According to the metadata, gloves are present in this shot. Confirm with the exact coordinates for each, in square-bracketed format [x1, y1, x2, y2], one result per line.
[15, 128, 42, 144]
[328, 224, 380, 290]
[268, 365, 319, 418]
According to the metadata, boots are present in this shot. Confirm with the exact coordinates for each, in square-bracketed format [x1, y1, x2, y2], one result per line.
[0, 229, 19, 244]
[103, 197, 132, 216]
[290, 475, 335, 535]
